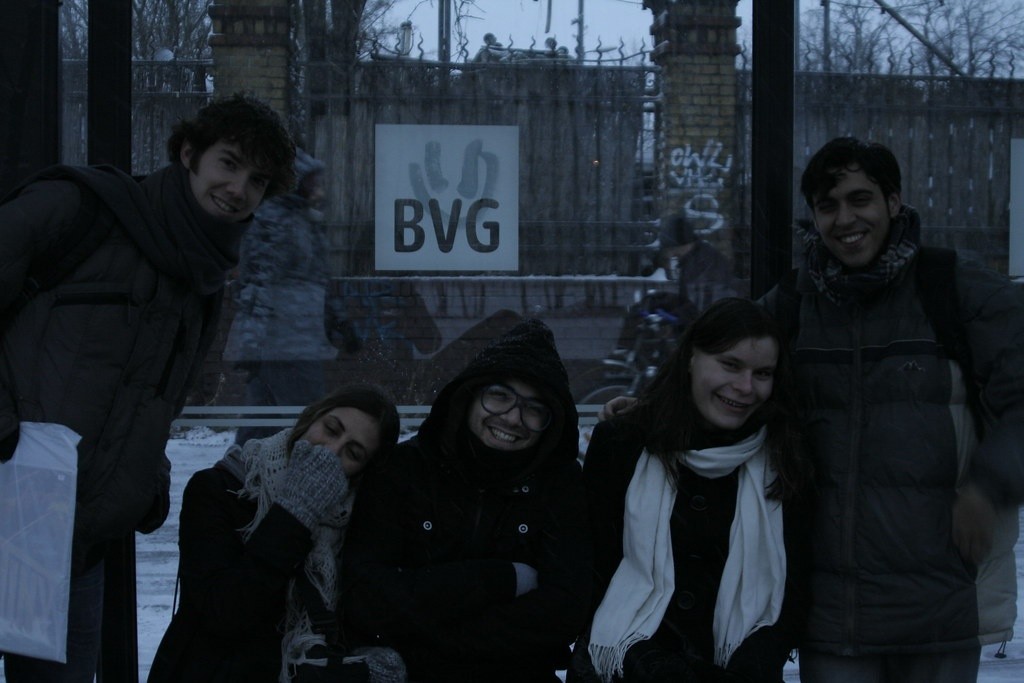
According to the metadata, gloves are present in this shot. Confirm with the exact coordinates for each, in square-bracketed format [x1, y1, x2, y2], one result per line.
[127, 471, 171, 534]
[274, 439, 349, 530]
[0, 420, 23, 463]
[352, 644, 408, 682]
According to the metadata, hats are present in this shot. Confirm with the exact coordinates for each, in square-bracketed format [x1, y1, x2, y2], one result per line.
[658, 213, 698, 245]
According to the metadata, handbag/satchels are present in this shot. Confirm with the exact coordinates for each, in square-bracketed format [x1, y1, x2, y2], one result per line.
[0, 420, 82, 663]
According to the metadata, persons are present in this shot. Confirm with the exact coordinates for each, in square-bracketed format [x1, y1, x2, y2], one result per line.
[603, 213, 746, 375]
[220, 145, 338, 443]
[595, 138, 1024, 683]
[0, 94, 298, 683]
[296, 319, 600, 683]
[565, 296, 826, 683]
[147, 384, 402, 683]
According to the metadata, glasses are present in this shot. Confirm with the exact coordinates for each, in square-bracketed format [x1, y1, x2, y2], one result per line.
[473, 380, 553, 433]
